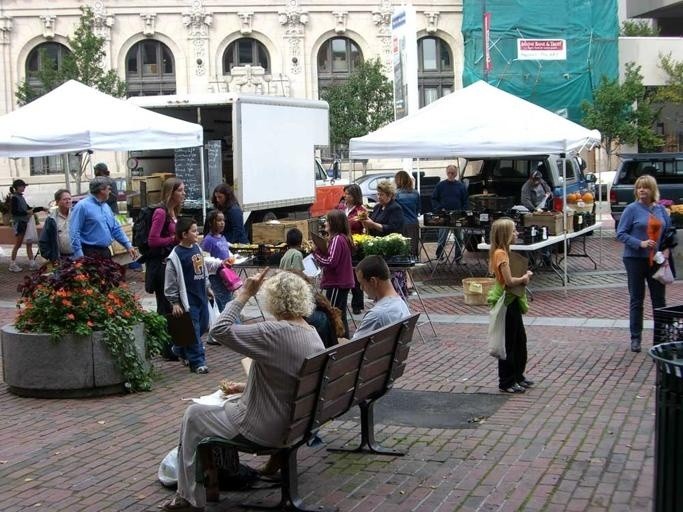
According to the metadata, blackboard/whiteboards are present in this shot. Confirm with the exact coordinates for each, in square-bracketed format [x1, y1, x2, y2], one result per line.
[174, 140, 223, 208]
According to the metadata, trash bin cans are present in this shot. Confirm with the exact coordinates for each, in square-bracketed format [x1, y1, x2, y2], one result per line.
[648, 341, 683, 512]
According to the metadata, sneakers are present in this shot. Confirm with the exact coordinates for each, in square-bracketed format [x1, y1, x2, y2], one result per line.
[29, 263, 38, 270]
[499, 381, 528, 393]
[631, 339, 641, 352]
[8, 264, 23, 272]
[196, 365, 209, 374]
[171, 346, 190, 366]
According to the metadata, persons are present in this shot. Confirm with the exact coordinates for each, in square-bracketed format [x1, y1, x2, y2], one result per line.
[9, 163, 551, 510]
[614, 173, 676, 353]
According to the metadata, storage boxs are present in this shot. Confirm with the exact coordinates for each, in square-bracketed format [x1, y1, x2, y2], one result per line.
[130, 171, 174, 210]
[524, 209, 572, 236]
[253, 219, 307, 245]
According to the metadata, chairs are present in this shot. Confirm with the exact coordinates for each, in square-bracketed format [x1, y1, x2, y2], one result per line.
[488, 167, 523, 177]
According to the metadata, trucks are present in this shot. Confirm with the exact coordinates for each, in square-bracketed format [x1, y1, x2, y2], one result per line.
[125, 94, 357, 237]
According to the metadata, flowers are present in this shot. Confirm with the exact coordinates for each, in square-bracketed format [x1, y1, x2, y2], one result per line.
[644, 214, 660, 266]
[13, 253, 173, 398]
[351, 232, 403, 263]
[656, 197, 682, 230]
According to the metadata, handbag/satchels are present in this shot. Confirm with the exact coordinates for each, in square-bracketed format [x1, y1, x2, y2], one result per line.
[219, 267, 243, 291]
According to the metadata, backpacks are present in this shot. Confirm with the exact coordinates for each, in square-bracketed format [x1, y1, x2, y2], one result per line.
[132, 205, 171, 250]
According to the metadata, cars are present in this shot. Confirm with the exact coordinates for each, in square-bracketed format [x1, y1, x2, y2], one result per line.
[48, 176, 127, 216]
[350, 172, 397, 202]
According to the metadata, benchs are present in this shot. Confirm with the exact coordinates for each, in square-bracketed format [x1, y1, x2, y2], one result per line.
[195, 313, 419, 512]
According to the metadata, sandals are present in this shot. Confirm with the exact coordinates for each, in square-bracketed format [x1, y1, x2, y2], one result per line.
[162, 496, 205, 512]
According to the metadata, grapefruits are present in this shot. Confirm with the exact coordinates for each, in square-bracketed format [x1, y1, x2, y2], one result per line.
[567, 192, 594, 202]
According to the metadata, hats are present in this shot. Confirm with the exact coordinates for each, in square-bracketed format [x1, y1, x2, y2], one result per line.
[13, 180, 28, 187]
[90, 176, 113, 193]
[377, 181, 396, 193]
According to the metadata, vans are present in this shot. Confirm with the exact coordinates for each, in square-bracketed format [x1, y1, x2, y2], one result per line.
[608, 153, 683, 232]
[457, 153, 598, 252]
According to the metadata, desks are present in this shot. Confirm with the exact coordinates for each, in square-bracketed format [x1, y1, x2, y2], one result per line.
[415, 218, 603, 300]
